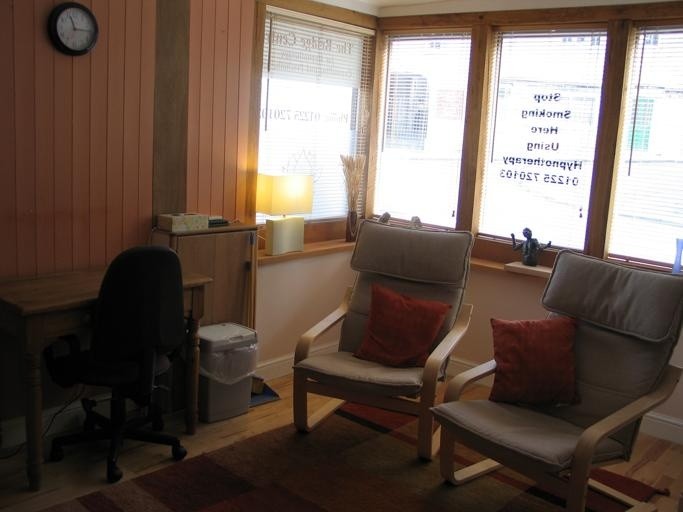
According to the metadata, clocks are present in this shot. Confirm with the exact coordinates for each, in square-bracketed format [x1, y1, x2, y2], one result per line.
[48, 1, 99, 58]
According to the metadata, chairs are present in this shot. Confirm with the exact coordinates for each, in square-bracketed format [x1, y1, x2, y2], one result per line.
[42, 245, 193, 484]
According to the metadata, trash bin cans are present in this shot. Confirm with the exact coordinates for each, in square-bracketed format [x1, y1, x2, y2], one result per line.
[197, 322, 259, 424]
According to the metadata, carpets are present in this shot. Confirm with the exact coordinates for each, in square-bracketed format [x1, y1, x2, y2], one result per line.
[37, 400, 670, 511]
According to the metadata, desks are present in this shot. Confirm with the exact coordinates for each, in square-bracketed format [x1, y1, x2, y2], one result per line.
[0, 266, 214, 494]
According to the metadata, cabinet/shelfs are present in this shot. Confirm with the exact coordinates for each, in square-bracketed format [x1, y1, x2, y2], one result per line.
[148, 223, 258, 329]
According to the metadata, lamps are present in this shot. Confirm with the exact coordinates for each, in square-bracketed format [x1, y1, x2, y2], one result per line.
[256, 169, 315, 256]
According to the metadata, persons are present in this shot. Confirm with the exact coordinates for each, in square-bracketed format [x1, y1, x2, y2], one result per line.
[510, 227, 550, 267]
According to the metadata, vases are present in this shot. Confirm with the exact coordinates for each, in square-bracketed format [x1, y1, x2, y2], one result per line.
[344, 211, 358, 242]
